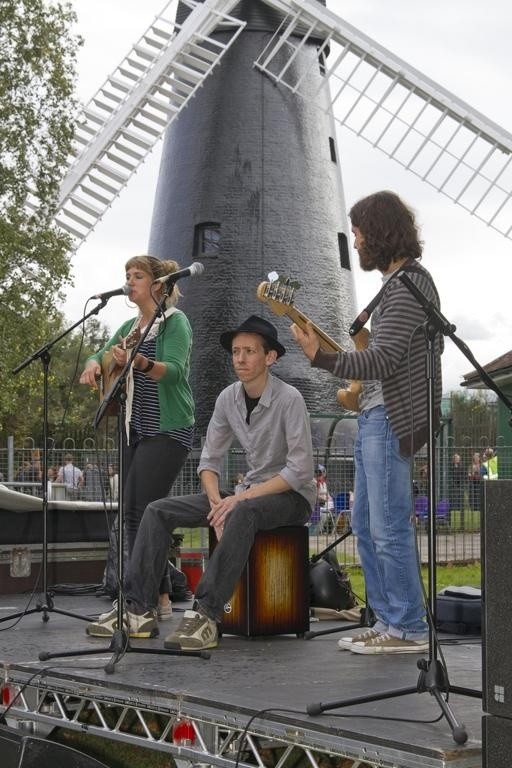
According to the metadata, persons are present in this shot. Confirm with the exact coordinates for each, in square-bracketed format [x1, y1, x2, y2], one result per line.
[78, 255, 195, 621]
[481, 447, 497, 480]
[48, 468, 57, 482]
[481, 451, 487, 462]
[86, 314, 318, 650]
[313, 467, 333, 512]
[449, 455, 461, 507]
[108, 465, 118, 501]
[290, 191, 443, 655]
[468, 452, 480, 510]
[56, 454, 82, 502]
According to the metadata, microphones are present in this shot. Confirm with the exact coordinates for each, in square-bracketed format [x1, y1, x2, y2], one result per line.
[89, 283, 133, 299]
[154, 260, 205, 285]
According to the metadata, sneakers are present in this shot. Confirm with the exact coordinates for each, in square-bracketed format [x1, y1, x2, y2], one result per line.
[98, 599, 118, 619]
[159, 601, 173, 621]
[85, 606, 160, 639]
[163, 610, 218, 650]
[351, 633, 429, 655]
[337, 628, 379, 650]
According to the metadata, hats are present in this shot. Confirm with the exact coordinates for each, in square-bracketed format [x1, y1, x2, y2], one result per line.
[219, 315, 286, 360]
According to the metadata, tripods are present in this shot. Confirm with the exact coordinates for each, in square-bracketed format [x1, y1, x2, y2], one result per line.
[0, 299, 108, 624]
[37, 284, 211, 675]
[306, 270, 512, 746]
[303, 529, 378, 641]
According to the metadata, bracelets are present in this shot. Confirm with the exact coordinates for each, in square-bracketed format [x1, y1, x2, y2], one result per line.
[142, 359, 154, 373]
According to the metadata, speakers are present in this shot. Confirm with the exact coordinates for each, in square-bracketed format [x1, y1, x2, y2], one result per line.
[480, 478, 512, 768]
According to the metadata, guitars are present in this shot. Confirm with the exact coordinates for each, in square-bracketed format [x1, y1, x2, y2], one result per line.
[99, 327, 142, 417]
[257, 270, 371, 413]
